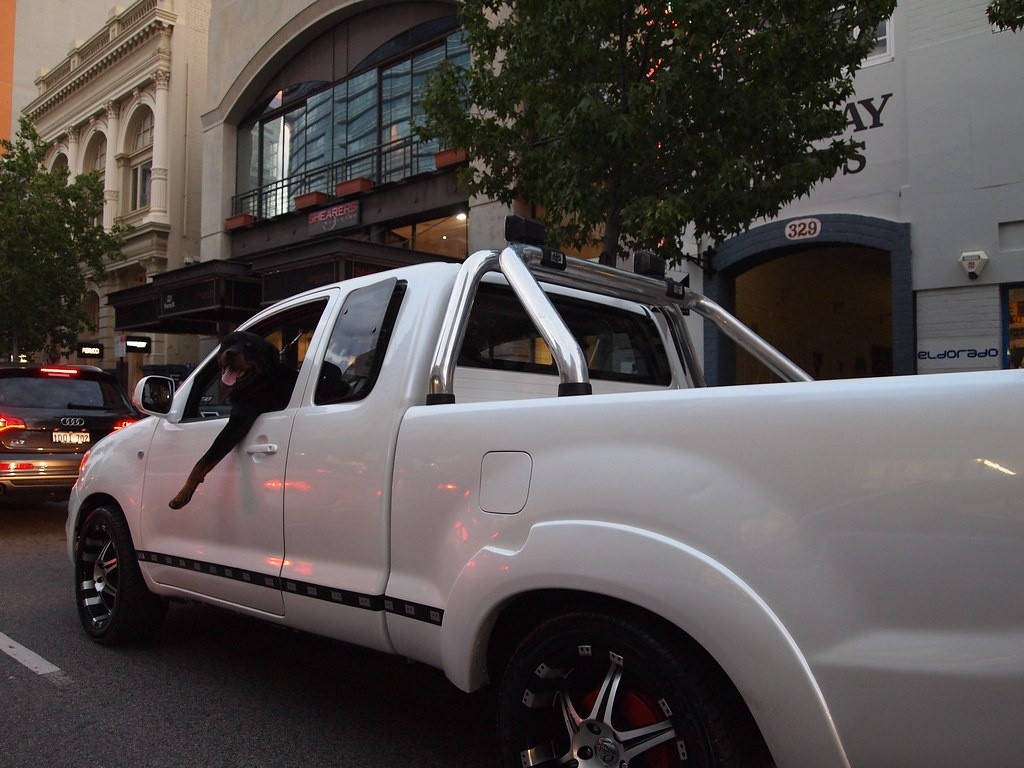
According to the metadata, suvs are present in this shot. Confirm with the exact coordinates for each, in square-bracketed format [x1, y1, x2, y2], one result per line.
[0, 363, 143, 493]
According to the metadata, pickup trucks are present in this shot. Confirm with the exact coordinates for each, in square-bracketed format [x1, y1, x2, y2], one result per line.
[64, 247, 1023, 768]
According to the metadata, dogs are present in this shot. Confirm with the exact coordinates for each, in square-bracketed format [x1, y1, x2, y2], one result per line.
[168, 330, 299, 510]
